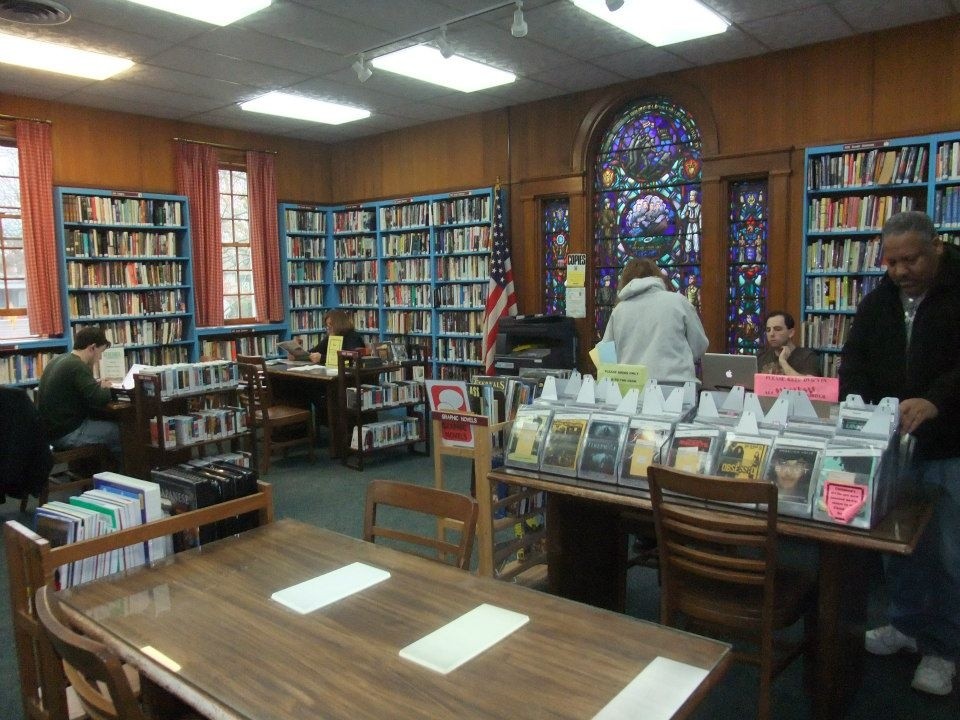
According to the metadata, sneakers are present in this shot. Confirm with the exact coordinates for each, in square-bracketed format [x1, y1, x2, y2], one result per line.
[864, 624, 918, 654]
[912, 655, 956, 695]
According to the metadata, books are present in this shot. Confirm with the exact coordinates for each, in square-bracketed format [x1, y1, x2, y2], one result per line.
[0, 194, 492, 591]
[804, 140, 960, 379]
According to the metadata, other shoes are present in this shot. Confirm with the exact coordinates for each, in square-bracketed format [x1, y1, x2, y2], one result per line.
[633, 538, 656, 552]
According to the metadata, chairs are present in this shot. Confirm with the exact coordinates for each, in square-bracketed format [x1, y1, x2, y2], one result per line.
[363, 479, 479, 569]
[237, 354, 296, 459]
[0, 384, 95, 511]
[34, 586, 146, 720]
[647, 464, 819, 720]
[237, 362, 317, 475]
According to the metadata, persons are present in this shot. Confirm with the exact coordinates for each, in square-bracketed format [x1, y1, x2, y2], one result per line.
[836, 211, 960, 696]
[37, 326, 126, 475]
[603, 257, 709, 566]
[288, 309, 365, 365]
[756, 312, 822, 378]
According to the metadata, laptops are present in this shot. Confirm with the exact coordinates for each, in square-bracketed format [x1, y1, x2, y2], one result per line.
[699, 352, 758, 392]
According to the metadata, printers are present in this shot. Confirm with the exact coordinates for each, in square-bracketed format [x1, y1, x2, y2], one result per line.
[494, 312, 577, 376]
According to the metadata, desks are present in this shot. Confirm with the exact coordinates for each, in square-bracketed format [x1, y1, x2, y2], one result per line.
[50, 517, 732, 720]
[487, 466, 942, 720]
[262, 361, 381, 461]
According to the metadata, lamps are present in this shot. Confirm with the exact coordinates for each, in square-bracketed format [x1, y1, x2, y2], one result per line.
[351, 52, 372, 82]
[510, 1, 528, 37]
[606, 0, 623, 12]
[434, 25, 455, 59]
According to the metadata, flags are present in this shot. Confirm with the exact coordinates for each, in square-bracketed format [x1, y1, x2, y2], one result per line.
[482, 182, 517, 377]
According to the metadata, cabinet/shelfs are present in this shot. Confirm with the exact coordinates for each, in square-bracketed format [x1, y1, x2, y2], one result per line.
[0, 131, 960, 720]
